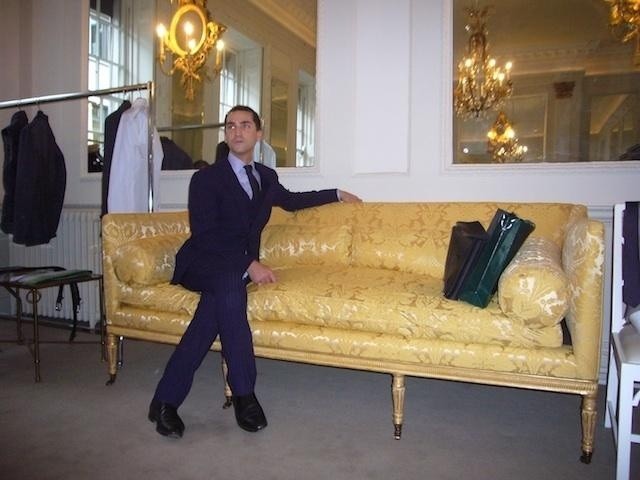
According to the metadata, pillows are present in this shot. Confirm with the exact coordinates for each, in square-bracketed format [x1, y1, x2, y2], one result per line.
[497, 236, 571, 329]
[258, 223, 353, 273]
[115, 231, 193, 289]
[352, 225, 451, 280]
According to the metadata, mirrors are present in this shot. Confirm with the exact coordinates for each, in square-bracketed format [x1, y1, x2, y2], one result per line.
[443, 0, 640, 172]
[81, 0, 324, 181]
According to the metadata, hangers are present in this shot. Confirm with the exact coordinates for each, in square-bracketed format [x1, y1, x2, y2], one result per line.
[35, 96, 45, 116]
[17, 97, 24, 112]
[135, 84, 146, 99]
[121, 86, 131, 102]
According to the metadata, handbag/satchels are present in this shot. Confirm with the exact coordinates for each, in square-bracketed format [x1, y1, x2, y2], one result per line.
[442, 208, 536, 310]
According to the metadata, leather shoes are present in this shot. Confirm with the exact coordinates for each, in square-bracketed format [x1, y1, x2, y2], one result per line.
[232, 392, 268, 433]
[147, 398, 185, 439]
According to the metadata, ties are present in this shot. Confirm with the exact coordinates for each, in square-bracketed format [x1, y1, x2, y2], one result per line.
[243, 164, 260, 201]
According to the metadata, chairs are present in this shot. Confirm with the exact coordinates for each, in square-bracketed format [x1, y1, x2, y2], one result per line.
[604, 202, 640, 480]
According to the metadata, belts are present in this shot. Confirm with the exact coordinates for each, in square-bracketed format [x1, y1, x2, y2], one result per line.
[0, 265, 82, 343]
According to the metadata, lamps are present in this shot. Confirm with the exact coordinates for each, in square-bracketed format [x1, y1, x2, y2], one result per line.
[488, 107, 528, 163]
[454, 7, 514, 122]
[155, 0, 227, 101]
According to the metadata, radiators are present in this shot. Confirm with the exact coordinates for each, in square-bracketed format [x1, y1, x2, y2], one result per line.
[9, 205, 104, 330]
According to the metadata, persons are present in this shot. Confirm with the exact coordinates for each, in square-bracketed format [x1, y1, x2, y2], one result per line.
[145, 103, 366, 445]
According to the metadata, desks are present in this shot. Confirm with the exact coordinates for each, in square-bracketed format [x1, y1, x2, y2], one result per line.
[0, 266, 104, 383]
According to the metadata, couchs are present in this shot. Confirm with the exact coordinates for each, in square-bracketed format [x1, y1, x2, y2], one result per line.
[101, 201, 605, 463]
